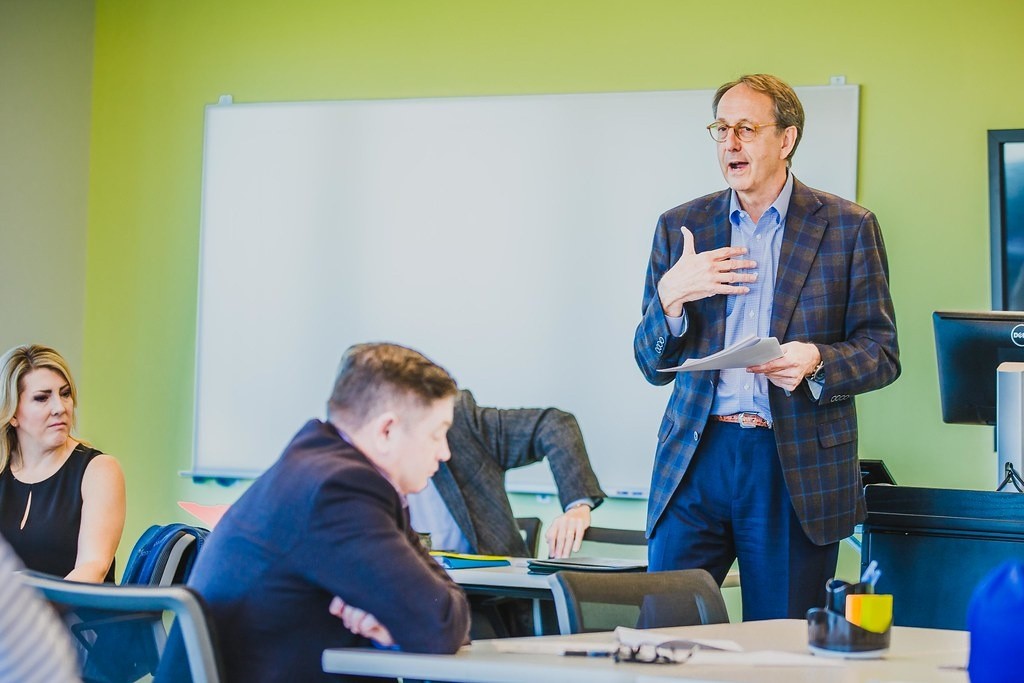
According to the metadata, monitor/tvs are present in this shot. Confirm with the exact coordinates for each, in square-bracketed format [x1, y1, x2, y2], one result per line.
[931, 310, 1024, 424]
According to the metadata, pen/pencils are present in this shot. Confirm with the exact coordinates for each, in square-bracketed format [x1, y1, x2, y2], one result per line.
[868, 569, 882, 587]
[860, 559, 878, 582]
[558, 649, 613, 656]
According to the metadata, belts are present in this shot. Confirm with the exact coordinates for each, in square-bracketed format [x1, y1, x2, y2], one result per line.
[707, 412, 774, 429]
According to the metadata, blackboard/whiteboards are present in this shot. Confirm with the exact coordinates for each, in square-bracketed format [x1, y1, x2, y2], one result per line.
[178, 79, 860, 504]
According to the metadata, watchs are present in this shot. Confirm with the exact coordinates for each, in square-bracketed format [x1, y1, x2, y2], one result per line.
[806, 361, 825, 382]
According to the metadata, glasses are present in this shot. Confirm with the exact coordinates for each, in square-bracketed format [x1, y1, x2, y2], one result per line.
[706, 121, 780, 142]
[612, 643, 699, 665]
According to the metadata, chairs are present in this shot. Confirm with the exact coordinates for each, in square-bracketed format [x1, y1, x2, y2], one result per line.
[23, 573, 219, 683]
[70, 525, 202, 683]
[518, 516, 743, 635]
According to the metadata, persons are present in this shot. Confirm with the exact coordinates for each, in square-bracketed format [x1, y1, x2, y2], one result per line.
[0, 346, 127, 586]
[154, 342, 472, 683]
[0, 536, 84, 683]
[407, 390, 607, 638]
[634, 73, 902, 630]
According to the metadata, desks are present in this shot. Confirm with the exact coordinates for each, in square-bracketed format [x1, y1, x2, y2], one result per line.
[322, 616, 996, 683]
[432, 552, 743, 601]
